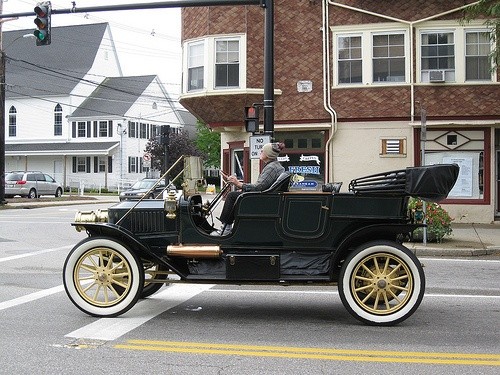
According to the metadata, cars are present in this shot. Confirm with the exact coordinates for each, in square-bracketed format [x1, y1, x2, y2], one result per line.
[119, 178, 174, 201]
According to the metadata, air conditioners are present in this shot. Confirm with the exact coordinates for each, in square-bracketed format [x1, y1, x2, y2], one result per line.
[427, 69, 445, 82]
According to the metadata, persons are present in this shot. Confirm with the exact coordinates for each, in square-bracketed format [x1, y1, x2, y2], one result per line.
[210, 142, 285, 237]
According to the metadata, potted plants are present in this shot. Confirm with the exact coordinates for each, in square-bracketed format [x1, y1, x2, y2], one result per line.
[408, 196, 454, 242]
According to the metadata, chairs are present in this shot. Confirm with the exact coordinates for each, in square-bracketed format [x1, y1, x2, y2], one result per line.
[350, 163, 460, 199]
[231, 172, 291, 216]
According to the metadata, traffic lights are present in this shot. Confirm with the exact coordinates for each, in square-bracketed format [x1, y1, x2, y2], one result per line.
[244, 105, 259, 133]
[33, 1, 51, 46]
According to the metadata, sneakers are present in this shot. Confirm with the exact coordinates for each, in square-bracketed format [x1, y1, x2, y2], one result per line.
[210, 228, 232, 237]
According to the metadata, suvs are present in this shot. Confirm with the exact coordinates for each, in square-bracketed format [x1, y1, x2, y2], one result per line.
[5, 169, 64, 200]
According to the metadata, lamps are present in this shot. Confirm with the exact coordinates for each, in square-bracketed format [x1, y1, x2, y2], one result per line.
[164, 190, 179, 220]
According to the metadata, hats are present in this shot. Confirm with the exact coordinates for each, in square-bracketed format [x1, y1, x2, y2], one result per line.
[263, 142, 285, 159]
